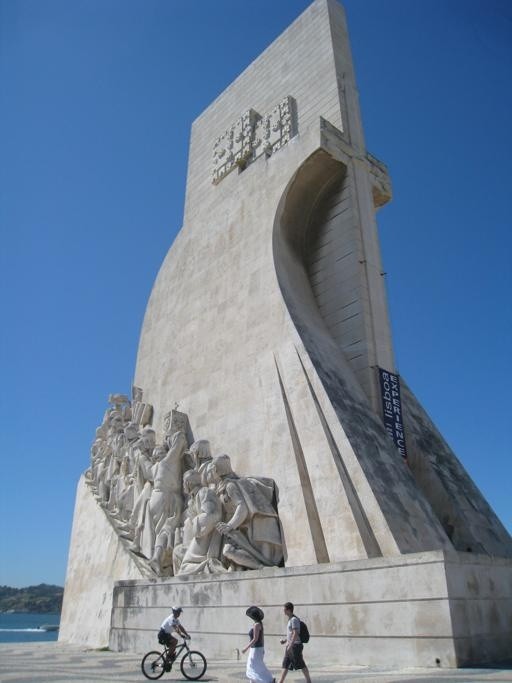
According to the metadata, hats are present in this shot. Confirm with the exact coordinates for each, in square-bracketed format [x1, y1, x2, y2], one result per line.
[171, 606, 183, 612]
[246, 606, 264, 621]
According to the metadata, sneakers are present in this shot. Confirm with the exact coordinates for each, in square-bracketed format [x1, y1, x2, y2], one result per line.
[166, 653, 175, 659]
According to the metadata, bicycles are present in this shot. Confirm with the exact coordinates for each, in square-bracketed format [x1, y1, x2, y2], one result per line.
[142, 636, 206, 681]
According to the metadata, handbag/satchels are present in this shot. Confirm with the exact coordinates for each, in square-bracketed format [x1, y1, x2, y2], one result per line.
[290, 615, 309, 644]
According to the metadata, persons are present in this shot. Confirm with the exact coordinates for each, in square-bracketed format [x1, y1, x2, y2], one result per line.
[278, 602, 312, 683]
[159, 605, 193, 670]
[85, 383, 285, 582]
[241, 605, 276, 683]
